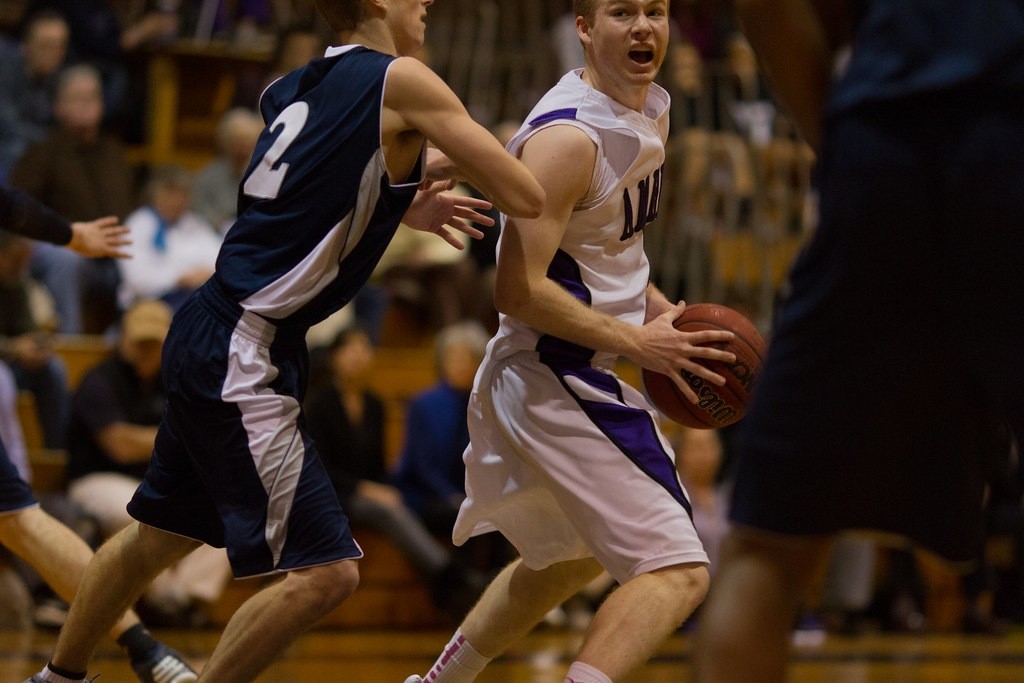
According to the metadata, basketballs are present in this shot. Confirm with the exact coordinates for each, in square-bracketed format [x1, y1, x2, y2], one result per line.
[643, 301, 768, 431]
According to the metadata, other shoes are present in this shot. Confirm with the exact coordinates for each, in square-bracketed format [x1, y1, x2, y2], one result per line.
[23, 672, 100, 683]
[133, 644, 200, 683]
[34, 600, 69, 628]
[428, 556, 485, 619]
[962, 613, 1009, 636]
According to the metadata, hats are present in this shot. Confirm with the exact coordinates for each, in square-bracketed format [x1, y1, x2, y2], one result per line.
[121, 300, 175, 346]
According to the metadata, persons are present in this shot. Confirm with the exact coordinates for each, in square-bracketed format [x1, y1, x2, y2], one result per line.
[0, 0, 1024, 683]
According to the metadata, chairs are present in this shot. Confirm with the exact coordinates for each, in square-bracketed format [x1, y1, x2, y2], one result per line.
[0, 21, 816, 604]
